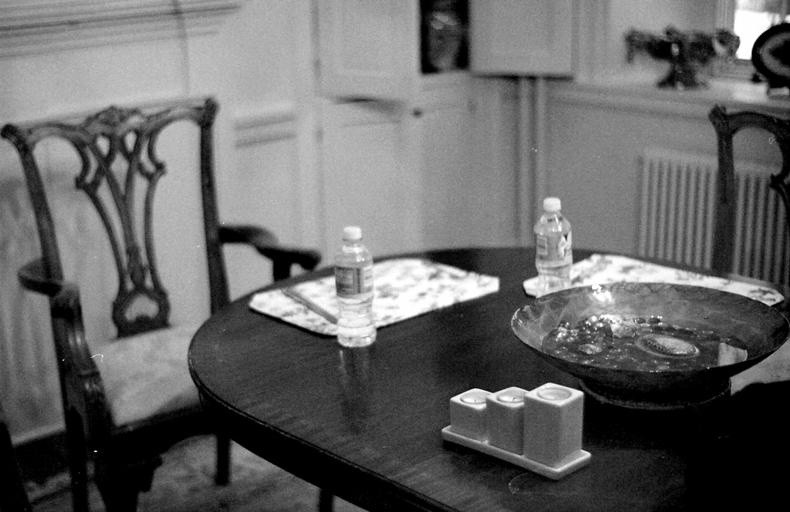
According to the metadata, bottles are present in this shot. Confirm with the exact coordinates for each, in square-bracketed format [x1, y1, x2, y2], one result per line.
[532, 197, 573, 300]
[335, 226, 377, 348]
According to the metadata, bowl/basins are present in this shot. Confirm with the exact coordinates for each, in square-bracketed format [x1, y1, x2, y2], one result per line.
[510, 281, 788, 408]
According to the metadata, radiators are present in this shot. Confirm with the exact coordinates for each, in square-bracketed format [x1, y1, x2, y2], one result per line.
[635, 147, 790, 285]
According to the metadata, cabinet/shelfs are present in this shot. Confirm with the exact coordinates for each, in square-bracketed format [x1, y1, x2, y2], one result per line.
[320, 78, 520, 263]
[317, 0, 573, 102]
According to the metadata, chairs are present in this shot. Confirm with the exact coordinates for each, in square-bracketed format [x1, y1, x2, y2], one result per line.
[2, 95, 333, 511]
[706, 101, 789, 285]
